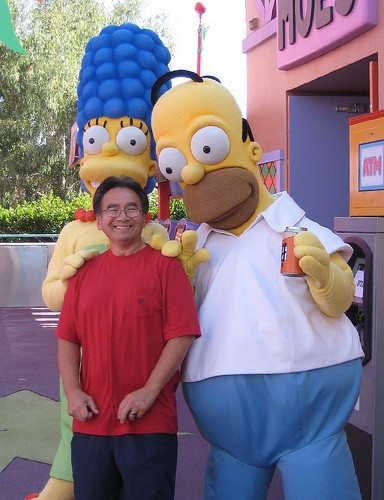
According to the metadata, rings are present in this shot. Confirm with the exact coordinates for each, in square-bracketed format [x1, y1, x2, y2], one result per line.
[130, 411, 136, 415]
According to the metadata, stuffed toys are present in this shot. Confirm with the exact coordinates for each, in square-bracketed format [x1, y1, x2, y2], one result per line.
[25, 24, 173, 500]
[149, 71, 366, 500]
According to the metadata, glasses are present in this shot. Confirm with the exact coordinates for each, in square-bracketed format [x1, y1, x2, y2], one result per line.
[95, 205, 146, 218]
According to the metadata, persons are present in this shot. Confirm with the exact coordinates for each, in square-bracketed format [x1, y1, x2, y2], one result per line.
[55, 174, 201, 500]
